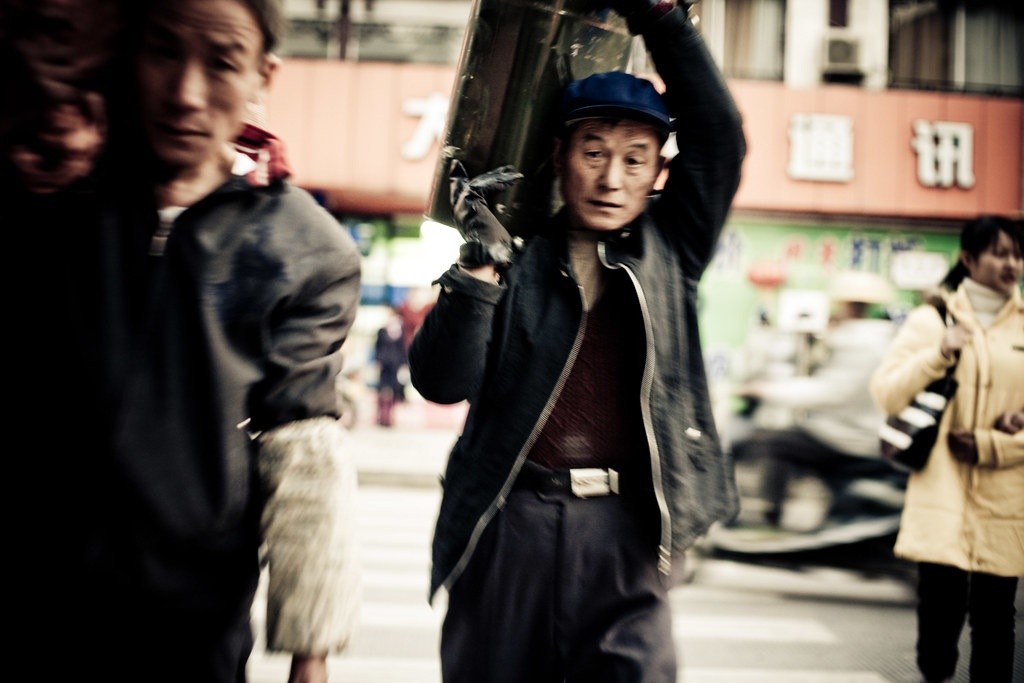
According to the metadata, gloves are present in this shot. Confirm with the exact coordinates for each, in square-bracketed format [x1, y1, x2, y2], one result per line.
[449, 161, 523, 273]
[949, 428, 976, 468]
[606, 0, 678, 40]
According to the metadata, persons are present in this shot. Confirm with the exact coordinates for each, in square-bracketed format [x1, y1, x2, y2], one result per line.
[1, 0, 367, 683]
[868, 212, 1024, 682]
[406, 4, 750, 683]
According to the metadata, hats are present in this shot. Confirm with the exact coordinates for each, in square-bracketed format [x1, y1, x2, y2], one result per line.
[562, 71, 671, 149]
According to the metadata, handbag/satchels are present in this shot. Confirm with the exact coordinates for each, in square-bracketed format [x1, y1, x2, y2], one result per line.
[878, 296, 958, 471]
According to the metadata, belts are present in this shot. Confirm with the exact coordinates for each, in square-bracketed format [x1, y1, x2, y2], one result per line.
[517, 467, 654, 499]
